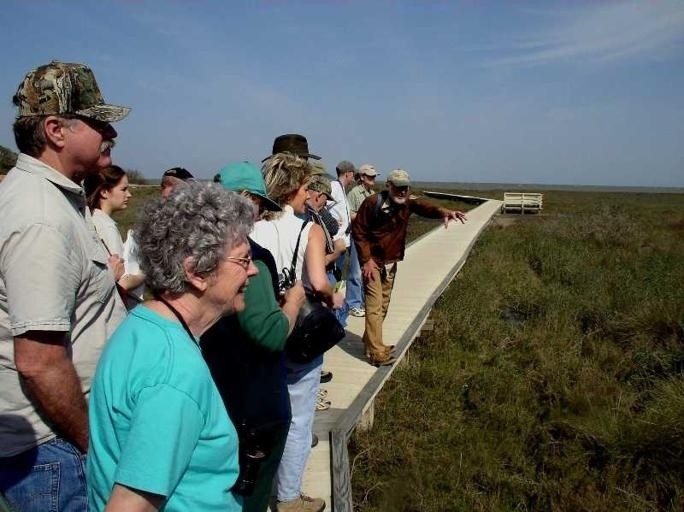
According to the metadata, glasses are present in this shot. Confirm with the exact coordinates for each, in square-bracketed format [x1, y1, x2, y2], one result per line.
[222, 255, 252, 269]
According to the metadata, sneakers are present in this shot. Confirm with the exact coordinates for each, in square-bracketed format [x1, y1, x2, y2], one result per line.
[277, 494, 326, 511]
[385, 344, 396, 353]
[347, 307, 364, 317]
[369, 353, 396, 364]
[316, 370, 332, 411]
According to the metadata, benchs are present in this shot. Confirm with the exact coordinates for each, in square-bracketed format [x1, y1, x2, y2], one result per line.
[502, 193, 542, 214]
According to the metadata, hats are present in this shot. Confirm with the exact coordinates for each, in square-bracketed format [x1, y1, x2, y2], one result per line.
[359, 163, 380, 175]
[308, 163, 337, 202]
[272, 135, 323, 161]
[387, 170, 412, 186]
[338, 160, 358, 173]
[311, 434, 318, 447]
[19, 61, 131, 121]
[163, 167, 193, 182]
[213, 163, 283, 212]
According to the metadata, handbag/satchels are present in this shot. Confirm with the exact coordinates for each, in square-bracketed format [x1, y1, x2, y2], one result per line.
[301, 308, 346, 356]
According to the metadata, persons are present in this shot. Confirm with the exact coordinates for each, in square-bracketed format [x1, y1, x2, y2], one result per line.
[2, 60, 137, 509]
[92, 133, 382, 512]
[351, 169, 469, 365]
